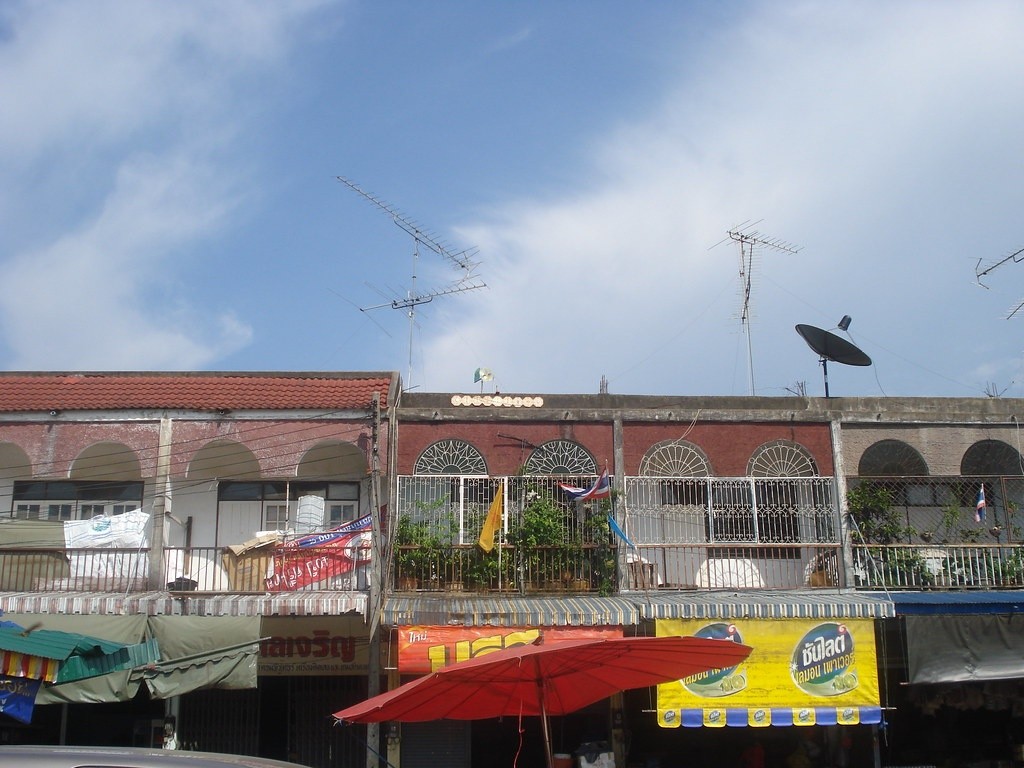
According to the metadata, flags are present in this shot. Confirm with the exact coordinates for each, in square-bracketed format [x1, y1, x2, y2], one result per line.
[266, 505, 386, 593]
[608, 518, 635, 547]
[560, 467, 609, 510]
[478, 481, 505, 555]
[976, 480, 989, 526]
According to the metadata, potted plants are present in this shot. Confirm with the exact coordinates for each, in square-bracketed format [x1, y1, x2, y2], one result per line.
[394, 464, 627, 597]
[847, 478, 1024, 591]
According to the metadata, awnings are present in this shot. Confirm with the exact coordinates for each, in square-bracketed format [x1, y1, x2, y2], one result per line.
[0, 590, 369, 725]
[382, 594, 1024, 727]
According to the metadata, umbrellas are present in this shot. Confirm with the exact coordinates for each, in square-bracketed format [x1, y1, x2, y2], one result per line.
[333, 635, 755, 768]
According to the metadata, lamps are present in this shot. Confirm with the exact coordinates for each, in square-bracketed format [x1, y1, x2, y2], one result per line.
[873, 732, 879, 744]
[611, 707, 633, 757]
[385, 720, 401, 746]
[163, 699, 176, 737]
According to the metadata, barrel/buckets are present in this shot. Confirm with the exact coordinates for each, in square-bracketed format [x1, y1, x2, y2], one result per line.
[553, 757, 577, 768]
[167, 577, 198, 592]
